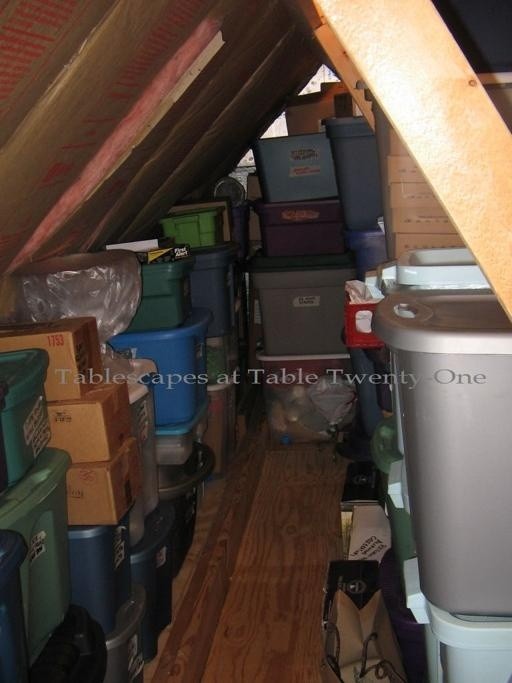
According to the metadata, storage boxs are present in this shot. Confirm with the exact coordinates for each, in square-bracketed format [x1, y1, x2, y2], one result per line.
[254, 351, 357, 445]
[0, 444, 70, 672]
[322, 115, 383, 231]
[157, 439, 216, 567]
[241, 268, 356, 356]
[0, 348, 51, 496]
[203, 388, 254, 477]
[252, 197, 346, 260]
[357, 73, 512, 262]
[122, 503, 181, 665]
[161, 205, 226, 253]
[402, 557, 512, 683]
[0, 531, 35, 683]
[284, 82, 360, 136]
[1, 313, 104, 405]
[65, 499, 136, 634]
[130, 504, 180, 661]
[41, 380, 131, 465]
[203, 328, 242, 387]
[105, 585, 148, 683]
[154, 404, 207, 465]
[227, 171, 263, 332]
[63, 437, 141, 527]
[108, 307, 210, 428]
[122, 253, 194, 335]
[374, 288, 512, 618]
[251, 132, 341, 201]
[188, 240, 242, 341]
[341, 265, 430, 681]
